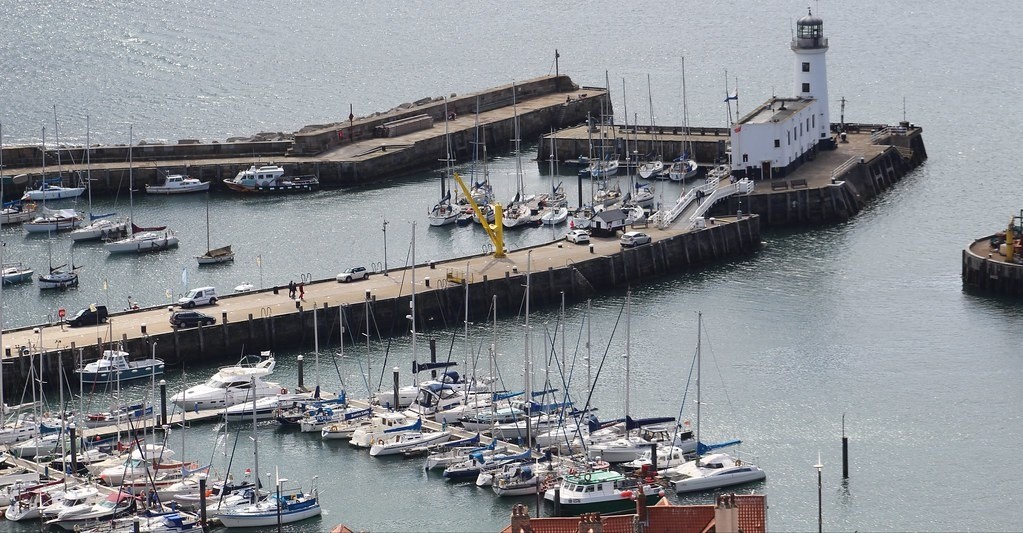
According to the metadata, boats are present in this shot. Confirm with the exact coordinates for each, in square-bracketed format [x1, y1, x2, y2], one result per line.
[73, 350, 166, 384]
[222, 145, 320, 194]
[144, 170, 210, 195]
[169, 351, 292, 413]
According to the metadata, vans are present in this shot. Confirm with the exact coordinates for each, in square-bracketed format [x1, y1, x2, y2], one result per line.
[177, 287, 218, 308]
[65, 305, 109, 327]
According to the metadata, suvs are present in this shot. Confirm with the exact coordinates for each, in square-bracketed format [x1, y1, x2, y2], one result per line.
[565, 229, 590, 244]
[336, 267, 369, 282]
[620, 231, 652, 247]
[169, 310, 216, 329]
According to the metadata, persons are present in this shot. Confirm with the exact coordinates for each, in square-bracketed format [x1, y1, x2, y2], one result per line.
[288, 280, 305, 299]
[696, 189, 705, 205]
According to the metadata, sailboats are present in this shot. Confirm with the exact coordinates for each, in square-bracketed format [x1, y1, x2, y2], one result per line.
[0, 221, 767, 533]
[195, 191, 236, 265]
[70, 113, 130, 242]
[428, 55, 699, 228]
[25, 125, 85, 233]
[0, 124, 37, 225]
[21, 102, 85, 202]
[103, 123, 179, 253]
